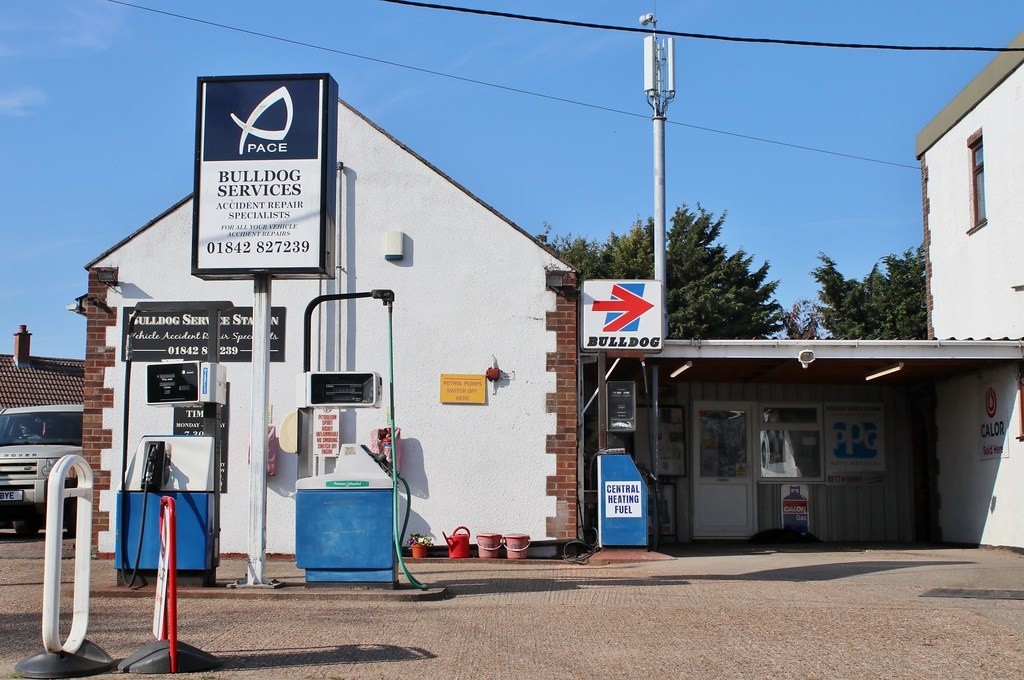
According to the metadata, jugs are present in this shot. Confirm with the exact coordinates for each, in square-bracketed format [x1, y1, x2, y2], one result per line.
[442, 526, 470, 558]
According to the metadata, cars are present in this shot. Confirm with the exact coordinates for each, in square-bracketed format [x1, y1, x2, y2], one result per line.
[3, 408, 82, 533]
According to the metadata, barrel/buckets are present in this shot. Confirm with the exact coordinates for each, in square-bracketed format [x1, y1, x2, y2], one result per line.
[502, 533, 531, 559]
[476, 533, 502, 558]
